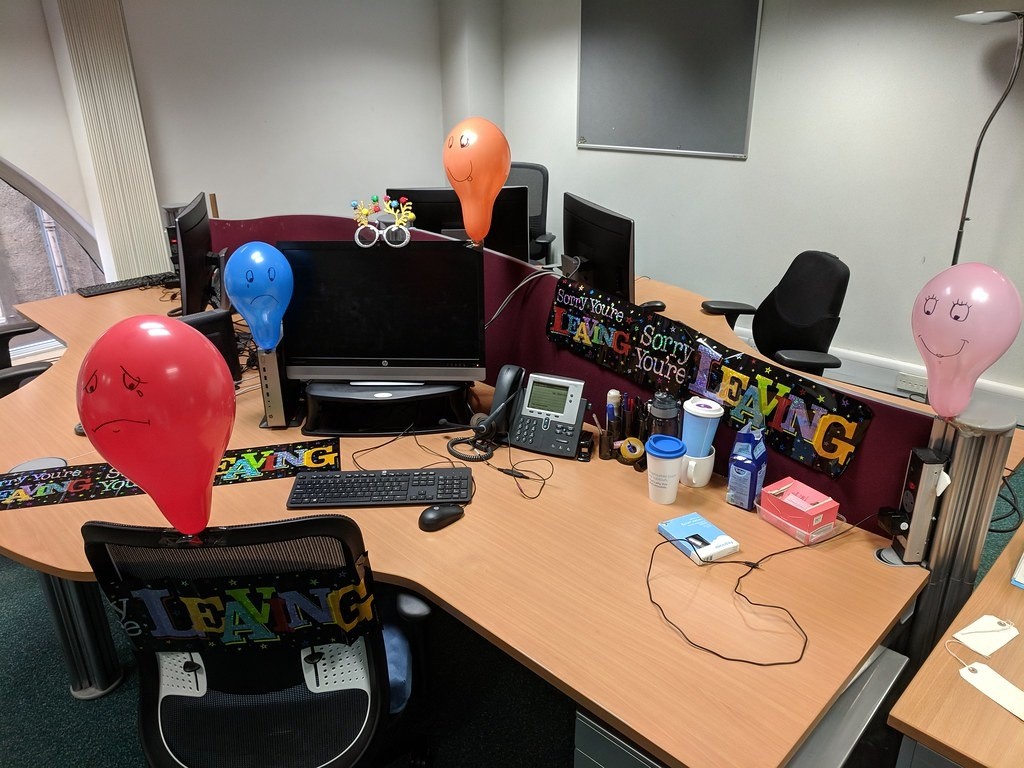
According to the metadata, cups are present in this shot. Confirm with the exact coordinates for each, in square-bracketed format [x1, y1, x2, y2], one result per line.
[680, 445, 715, 487]
[598, 409, 649, 465]
[644, 435, 687, 505]
[682, 396, 724, 456]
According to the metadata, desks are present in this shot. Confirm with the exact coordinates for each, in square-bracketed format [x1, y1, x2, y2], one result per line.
[0, 215, 1024, 768]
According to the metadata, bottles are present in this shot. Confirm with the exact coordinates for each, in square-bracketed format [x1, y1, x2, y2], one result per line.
[649, 400, 678, 439]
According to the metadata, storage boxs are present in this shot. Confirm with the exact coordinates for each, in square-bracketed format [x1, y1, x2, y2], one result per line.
[657, 513, 739, 567]
[762, 478, 839, 543]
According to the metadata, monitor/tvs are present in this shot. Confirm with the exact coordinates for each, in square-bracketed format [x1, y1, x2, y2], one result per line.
[276, 239, 486, 401]
[174, 192, 230, 316]
[386, 185, 530, 264]
[560, 193, 635, 304]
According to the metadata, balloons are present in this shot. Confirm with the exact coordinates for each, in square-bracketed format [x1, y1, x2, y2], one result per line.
[443, 117, 511, 246]
[912, 261, 1024, 420]
[76, 314, 236, 544]
[224, 241, 294, 355]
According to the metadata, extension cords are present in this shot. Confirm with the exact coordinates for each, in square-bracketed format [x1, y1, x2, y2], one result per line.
[891, 445, 947, 564]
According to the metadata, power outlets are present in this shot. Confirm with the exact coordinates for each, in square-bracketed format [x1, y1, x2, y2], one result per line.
[896, 372, 929, 395]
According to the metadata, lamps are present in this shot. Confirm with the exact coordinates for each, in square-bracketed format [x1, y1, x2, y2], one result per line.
[925, 12, 1024, 405]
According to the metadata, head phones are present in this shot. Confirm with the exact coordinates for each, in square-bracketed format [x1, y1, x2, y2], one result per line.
[470, 386, 524, 439]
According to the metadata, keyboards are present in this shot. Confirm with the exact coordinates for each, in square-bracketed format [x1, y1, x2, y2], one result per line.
[286, 467, 472, 506]
[76, 272, 177, 298]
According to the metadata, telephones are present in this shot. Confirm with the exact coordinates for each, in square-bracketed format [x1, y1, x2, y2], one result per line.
[483, 363, 588, 460]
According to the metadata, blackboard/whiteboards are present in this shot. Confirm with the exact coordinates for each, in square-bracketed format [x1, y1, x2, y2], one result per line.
[575, 0, 764, 161]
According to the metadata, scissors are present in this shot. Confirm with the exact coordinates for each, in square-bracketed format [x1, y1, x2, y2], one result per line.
[635, 395, 648, 439]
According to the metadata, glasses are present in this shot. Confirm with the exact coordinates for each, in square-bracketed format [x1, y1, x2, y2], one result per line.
[350, 196, 416, 248]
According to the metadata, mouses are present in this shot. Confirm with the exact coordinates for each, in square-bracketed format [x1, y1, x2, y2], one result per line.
[419, 503, 464, 532]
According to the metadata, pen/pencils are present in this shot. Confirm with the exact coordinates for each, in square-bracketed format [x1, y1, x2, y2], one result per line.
[593, 389, 654, 435]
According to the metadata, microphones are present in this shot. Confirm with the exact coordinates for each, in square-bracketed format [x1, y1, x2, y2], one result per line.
[439, 419, 485, 432]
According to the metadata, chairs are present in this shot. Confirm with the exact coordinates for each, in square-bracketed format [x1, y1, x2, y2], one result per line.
[82, 512, 431, 768]
[700, 251, 851, 376]
[499, 162, 556, 264]
[0, 322, 52, 399]
